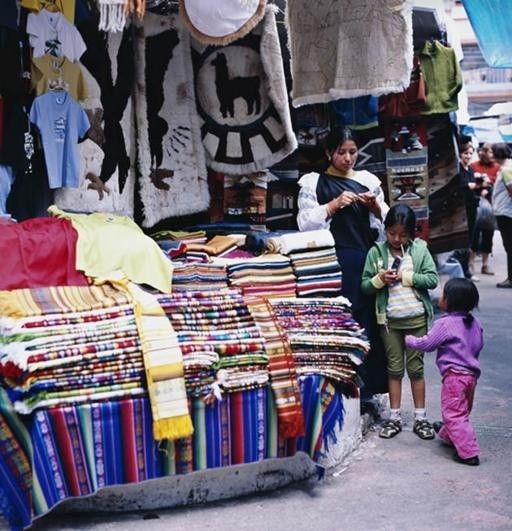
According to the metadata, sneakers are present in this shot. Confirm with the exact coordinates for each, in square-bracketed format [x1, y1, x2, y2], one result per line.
[454, 451, 479, 465]
[433, 420, 445, 433]
[467, 275, 482, 282]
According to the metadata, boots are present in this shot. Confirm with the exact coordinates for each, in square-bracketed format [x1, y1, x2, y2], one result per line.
[481, 266, 495, 276]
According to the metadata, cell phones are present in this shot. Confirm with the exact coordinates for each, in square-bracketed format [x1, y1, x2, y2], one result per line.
[391, 256, 403, 274]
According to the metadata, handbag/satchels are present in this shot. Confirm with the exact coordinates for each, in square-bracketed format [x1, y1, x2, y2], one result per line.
[476, 196, 498, 231]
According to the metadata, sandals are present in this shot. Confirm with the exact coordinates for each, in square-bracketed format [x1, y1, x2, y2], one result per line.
[379, 419, 402, 439]
[413, 420, 435, 440]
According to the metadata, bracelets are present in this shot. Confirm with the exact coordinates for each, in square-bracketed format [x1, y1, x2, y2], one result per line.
[324, 200, 336, 216]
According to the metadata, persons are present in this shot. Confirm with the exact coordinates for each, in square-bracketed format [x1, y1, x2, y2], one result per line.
[360, 205, 434, 440]
[466, 142, 499, 276]
[295, 125, 395, 425]
[445, 140, 479, 285]
[404, 277, 484, 468]
[489, 143, 511, 289]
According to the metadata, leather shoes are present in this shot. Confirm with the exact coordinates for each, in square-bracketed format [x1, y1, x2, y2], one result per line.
[497, 279, 512, 288]
